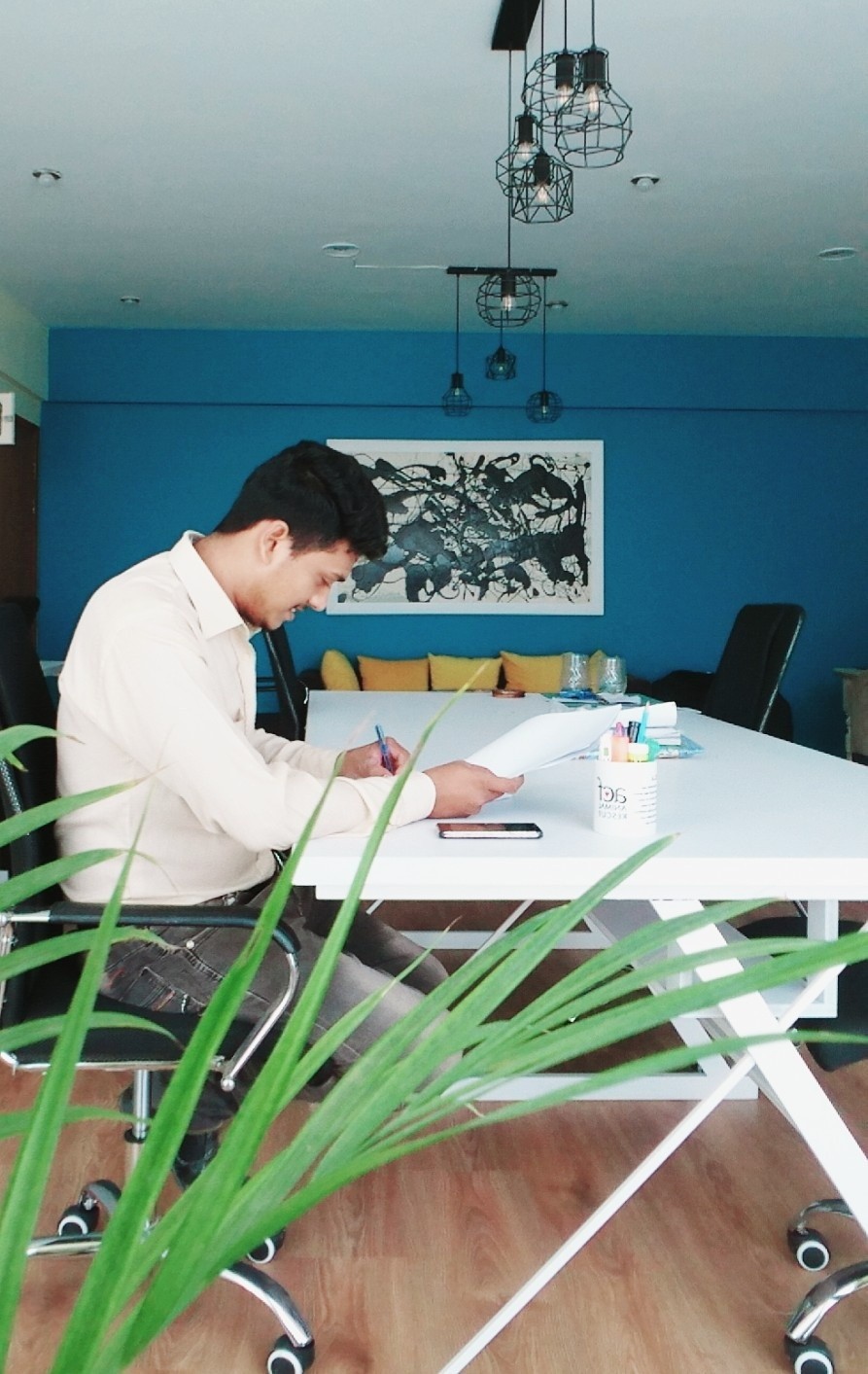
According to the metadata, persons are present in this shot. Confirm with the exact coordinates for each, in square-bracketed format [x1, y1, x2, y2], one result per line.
[57, 438, 527, 1193]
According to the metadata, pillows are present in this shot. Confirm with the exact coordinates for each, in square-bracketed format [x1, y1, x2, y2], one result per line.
[356, 655, 428, 692]
[319, 650, 361, 692]
[587, 650, 610, 695]
[426, 651, 503, 692]
[501, 651, 563, 693]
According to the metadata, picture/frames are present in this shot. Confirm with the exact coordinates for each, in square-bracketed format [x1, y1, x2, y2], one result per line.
[326, 438, 604, 616]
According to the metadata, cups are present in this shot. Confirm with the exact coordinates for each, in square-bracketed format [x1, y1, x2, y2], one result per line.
[561, 653, 591, 696]
[593, 760, 657, 836]
[596, 656, 627, 693]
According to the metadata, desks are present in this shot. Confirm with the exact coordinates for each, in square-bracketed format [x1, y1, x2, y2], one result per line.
[290, 691, 868, 1374]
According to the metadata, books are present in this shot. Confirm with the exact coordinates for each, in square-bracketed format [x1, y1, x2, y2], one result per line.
[539, 690, 707, 760]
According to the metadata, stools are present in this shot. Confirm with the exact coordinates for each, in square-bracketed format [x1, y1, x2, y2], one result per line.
[735, 899, 868, 1374]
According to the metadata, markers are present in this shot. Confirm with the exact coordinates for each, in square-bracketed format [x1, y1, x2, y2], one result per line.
[628, 721, 641, 744]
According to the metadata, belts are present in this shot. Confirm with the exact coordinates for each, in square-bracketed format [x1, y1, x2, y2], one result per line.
[221, 848, 288, 906]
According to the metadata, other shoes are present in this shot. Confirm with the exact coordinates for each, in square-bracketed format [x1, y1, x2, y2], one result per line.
[117, 1086, 251, 1199]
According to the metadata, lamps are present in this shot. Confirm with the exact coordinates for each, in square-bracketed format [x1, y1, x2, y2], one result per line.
[443, 266, 558, 427]
[476, 1, 632, 329]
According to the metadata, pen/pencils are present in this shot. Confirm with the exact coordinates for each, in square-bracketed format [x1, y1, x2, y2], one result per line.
[616, 723, 625, 736]
[637, 701, 651, 743]
[375, 724, 392, 774]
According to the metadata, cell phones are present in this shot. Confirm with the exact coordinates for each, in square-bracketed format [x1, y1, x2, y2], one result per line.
[437, 822, 544, 837]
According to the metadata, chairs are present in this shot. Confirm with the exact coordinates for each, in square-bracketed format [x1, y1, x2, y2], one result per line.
[256, 622, 309, 743]
[0, 595, 335, 1374]
[699, 603, 806, 734]
